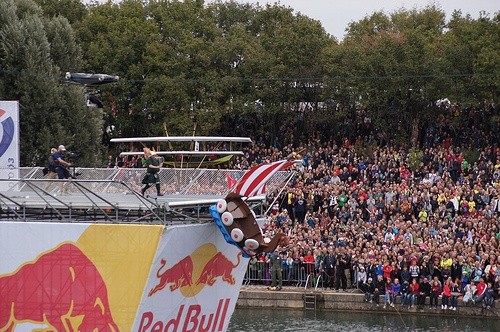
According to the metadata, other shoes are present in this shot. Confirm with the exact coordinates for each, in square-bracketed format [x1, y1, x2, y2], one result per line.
[420, 304, 424, 309]
[416, 305, 420, 310]
[400, 303, 404, 306]
[404, 306, 406, 309]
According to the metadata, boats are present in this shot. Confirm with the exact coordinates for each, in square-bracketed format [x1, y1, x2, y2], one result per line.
[0, 157, 298, 332]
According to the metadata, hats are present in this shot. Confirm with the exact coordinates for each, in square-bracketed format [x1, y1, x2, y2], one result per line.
[59, 145, 66, 151]
[149, 151, 156, 157]
[51, 148, 57, 153]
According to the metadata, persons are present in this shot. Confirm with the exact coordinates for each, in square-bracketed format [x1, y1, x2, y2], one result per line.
[107, 117, 500, 312]
[40, 147, 59, 194]
[54, 144, 72, 195]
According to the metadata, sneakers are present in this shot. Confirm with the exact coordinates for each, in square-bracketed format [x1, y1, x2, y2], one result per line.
[411, 305, 414, 308]
[487, 306, 492, 310]
[482, 303, 486, 308]
[365, 298, 394, 308]
[429, 305, 432, 309]
[141, 189, 145, 195]
[453, 307, 457, 310]
[449, 306, 453, 310]
[157, 193, 164, 196]
[441, 305, 444, 309]
[444, 305, 447, 309]
[433, 305, 437, 309]
[408, 306, 410, 309]
[462, 302, 466, 306]
[472, 299, 475, 305]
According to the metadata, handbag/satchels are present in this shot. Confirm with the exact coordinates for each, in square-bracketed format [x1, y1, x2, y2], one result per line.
[43, 168, 48, 176]
